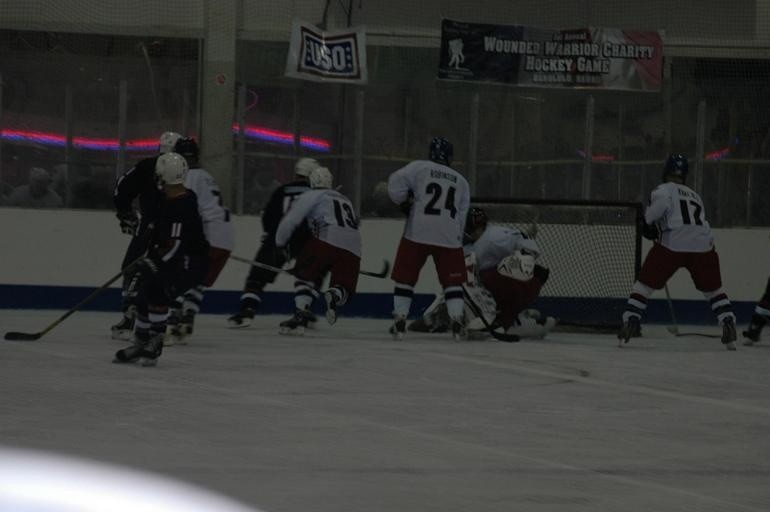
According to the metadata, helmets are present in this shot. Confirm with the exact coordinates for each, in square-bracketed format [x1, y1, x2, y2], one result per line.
[429, 137, 453, 164]
[295, 157, 319, 178]
[461, 208, 488, 246]
[309, 168, 333, 188]
[156, 152, 188, 184]
[175, 137, 198, 161]
[159, 132, 183, 154]
[663, 152, 689, 181]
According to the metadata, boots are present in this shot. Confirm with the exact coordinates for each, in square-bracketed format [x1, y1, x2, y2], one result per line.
[445, 287, 465, 338]
[389, 296, 412, 334]
[326, 287, 346, 324]
[112, 289, 204, 361]
[742, 289, 770, 342]
[280, 296, 310, 327]
[703, 289, 737, 344]
[228, 294, 260, 325]
[616, 292, 648, 342]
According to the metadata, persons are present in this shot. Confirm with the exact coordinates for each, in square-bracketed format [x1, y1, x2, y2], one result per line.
[110, 130, 187, 332]
[165, 136, 235, 339]
[388, 137, 472, 335]
[407, 205, 557, 341]
[617, 151, 738, 343]
[275, 166, 367, 331]
[116, 151, 214, 368]
[227, 156, 324, 323]
[7, 161, 65, 208]
[742, 274, 770, 343]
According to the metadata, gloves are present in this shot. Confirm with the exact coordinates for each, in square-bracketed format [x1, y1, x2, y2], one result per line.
[119, 214, 141, 236]
[638, 215, 660, 241]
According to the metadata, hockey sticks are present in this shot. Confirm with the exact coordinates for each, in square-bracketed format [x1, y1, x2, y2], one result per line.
[664, 280, 681, 338]
[462, 284, 522, 342]
[3, 246, 150, 342]
[359, 257, 391, 279]
